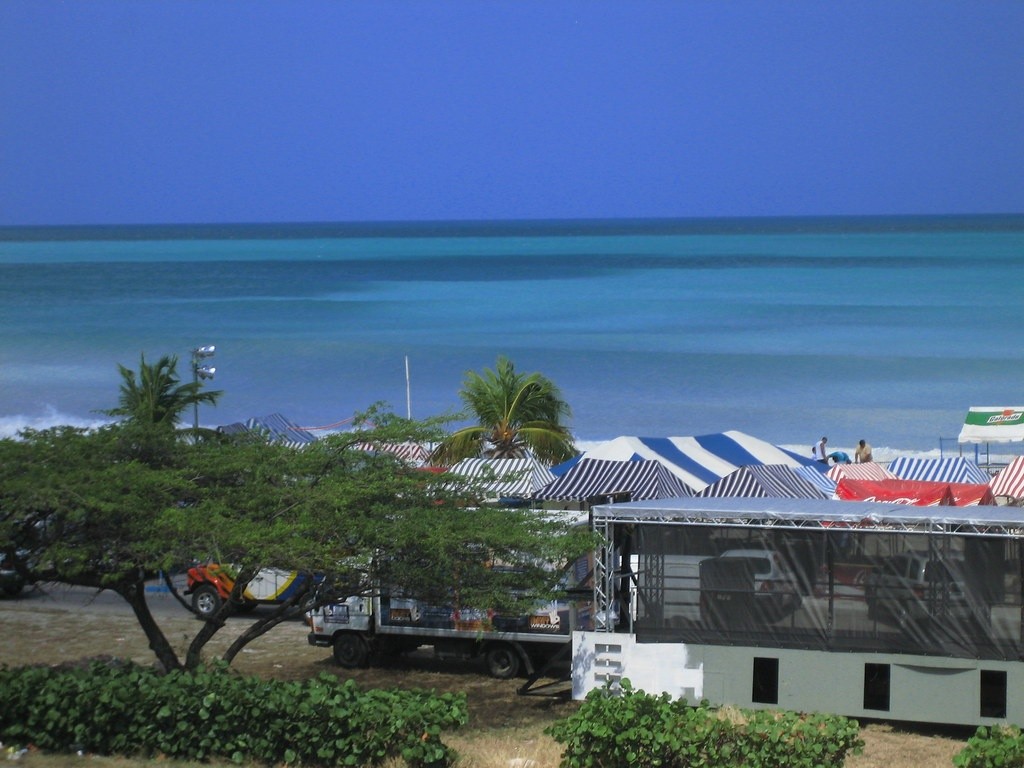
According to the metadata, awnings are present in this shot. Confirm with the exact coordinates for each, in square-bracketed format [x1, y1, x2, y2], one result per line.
[958, 406, 1024, 443]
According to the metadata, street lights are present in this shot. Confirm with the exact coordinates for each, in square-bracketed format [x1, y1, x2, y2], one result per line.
[191, 336, 219, 426]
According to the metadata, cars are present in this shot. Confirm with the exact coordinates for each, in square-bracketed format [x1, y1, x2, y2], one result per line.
[865, 552, 974, 636]
[720, 548, 803, 621]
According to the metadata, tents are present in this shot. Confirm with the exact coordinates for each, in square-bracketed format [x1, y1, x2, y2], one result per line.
[215, 412, 1024, 506]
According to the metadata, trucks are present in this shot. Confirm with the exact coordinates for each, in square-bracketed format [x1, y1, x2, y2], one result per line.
[300, 508, 594, 681]
[184, 552, 316, 624]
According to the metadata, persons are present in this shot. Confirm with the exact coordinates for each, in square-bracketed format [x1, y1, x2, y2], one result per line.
[816, 437, 827, 463]
[825, 451, 851, 463]
[854, 439, 873, 463]
[811, 447, 816, 459]
